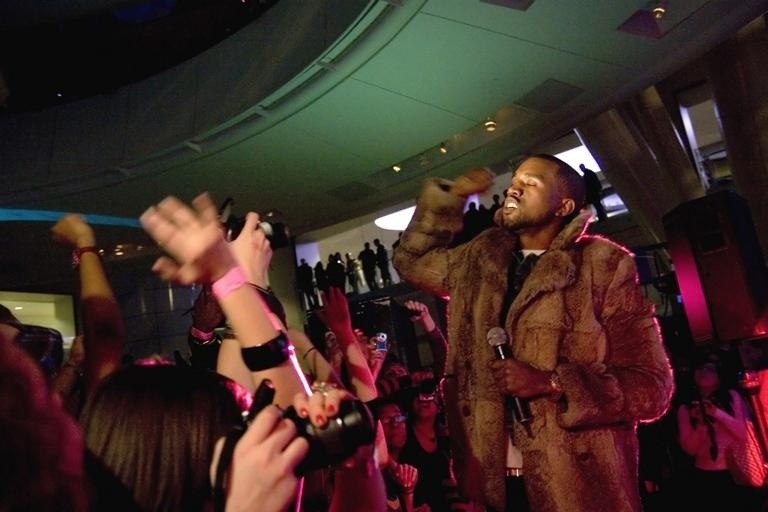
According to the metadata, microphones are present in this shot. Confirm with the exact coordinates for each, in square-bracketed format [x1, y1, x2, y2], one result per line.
[487, 327, 532, 423]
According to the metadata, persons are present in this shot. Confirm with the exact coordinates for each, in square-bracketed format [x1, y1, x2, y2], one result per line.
[461, 186, 508, 241]
[1, 190, 473, 512]
[390, 149, 674, 512]
[577, 162, 610, 223]
[674, 355, 747, 511]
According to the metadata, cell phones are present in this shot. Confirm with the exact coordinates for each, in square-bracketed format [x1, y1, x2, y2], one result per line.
[376, 333, 387, 351]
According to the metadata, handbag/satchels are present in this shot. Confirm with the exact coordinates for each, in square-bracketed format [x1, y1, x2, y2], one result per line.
[732, 418, 765, 487]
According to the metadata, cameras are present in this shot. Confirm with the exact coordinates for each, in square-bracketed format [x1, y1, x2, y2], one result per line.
[226, 216, 291, 250]
[420, 380, 435, 394]
[269, 400, 375, 479]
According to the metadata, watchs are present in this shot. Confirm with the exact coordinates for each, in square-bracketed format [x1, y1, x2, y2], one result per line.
[546, 370, 563, 404]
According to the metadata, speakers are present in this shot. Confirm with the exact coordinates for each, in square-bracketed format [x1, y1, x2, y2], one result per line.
[663, 192, 768, 343]
